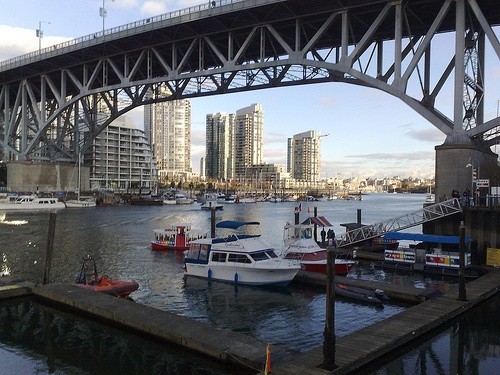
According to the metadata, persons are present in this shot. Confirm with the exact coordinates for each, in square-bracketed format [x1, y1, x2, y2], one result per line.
[463, 188, 471, 206]
[327, 229, 332, 244]
[331, 230, 335, 244]
[321, 228, 326, 243]
[452, 191, 461, 208]
[474, 189, 481, 206]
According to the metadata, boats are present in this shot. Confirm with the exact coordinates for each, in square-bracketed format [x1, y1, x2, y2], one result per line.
[182, 221, 301, 286]
[0, 153, 363, 210]
[425, 184, 434, 202]
[151, 225, 208, 251]
[340, 208, 400, 251]
[75, 255, 139, 299]
[279, 221, 356, 276]
